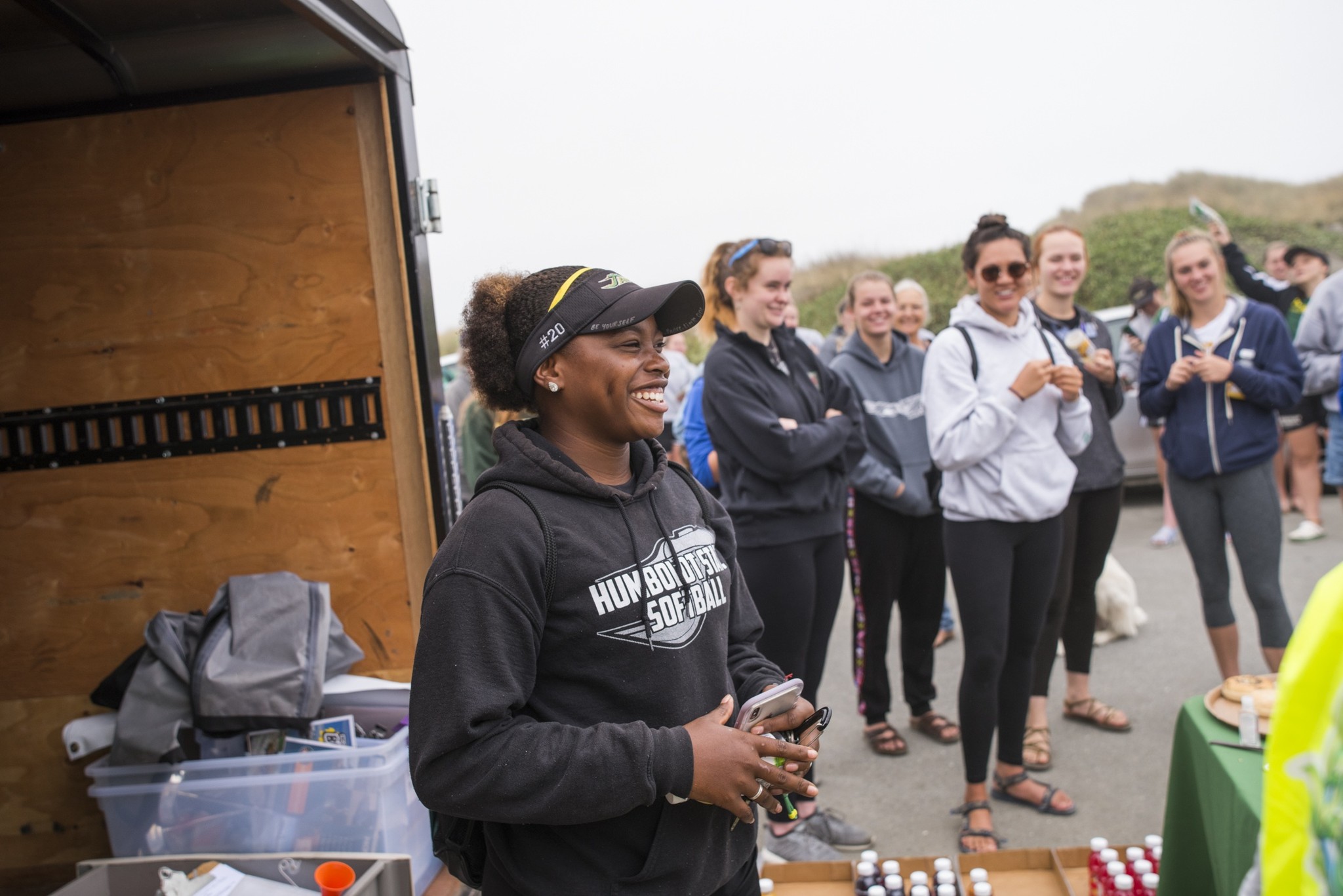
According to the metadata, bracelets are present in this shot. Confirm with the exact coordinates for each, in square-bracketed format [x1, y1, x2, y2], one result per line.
[1010, 387, 1026, 402]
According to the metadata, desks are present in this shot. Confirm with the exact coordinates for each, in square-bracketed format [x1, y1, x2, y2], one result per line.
[1156, 696, 1265, 896]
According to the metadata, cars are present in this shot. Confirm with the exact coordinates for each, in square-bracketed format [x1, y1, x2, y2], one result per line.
[1092, 302, 1169, 492]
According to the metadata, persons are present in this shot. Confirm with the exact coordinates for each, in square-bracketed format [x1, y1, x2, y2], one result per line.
[703, 237, 876, 865]
[922, 214, 1093, 854]
[1115, 280, 1185, 548]
[1021, 223, 1131, 770]
[655, 268, 961, 760]
[455, 390, 540, 512]
[406, 263, 819, 895]
[1211, 222, 1343, 539]
[1139, 226, 1306, 681]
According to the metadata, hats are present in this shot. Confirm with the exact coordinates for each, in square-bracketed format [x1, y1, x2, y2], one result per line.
[1129, 279, 1158, 308]
[516, 271, 705, 393]
[1283, 245, 1330, 267]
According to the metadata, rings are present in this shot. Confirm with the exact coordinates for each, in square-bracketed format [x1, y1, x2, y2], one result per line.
[747, 781, 763, 800]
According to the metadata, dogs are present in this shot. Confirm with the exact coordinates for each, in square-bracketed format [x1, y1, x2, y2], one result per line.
[1051, 549, 1149, 658]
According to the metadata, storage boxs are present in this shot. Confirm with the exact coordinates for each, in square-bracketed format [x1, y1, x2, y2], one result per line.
[85, 726, 444, 896]
[759, 840, 1146, 896]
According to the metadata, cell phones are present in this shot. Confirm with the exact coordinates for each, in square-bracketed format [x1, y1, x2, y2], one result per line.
[734, 678, 805, 734]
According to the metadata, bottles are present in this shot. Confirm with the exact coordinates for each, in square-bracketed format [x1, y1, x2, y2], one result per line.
[1224, 348, 1256, 399]
[1087, 834, 1163, 896]
[854, 851, 993, 896]
[1064, 329, 1101, 363]
[759, 878, 777, 896]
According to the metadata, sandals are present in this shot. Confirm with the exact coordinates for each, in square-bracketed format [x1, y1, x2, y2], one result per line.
[950, 801, 1004, 852]
[863, 723, 907, 754]
[933, 627, 954, 646]
[1063, 697, 1132, 732]
[1022, 720, 1052, 770]
[990, 769, 1077, 815]
[912, 713, 960, 744]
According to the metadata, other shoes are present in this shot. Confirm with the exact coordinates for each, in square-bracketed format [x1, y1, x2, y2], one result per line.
[1289, 520, 1329, 541]
[1152, 527, 1181, 543]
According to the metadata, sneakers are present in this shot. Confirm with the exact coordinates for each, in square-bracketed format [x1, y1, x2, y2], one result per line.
[761, 822, 847, 864]
[800, 806, 873, 850]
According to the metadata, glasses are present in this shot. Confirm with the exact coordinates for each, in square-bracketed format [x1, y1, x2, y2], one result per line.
[728, 238, 793, 266]
[980, 261, 1030, 282]
